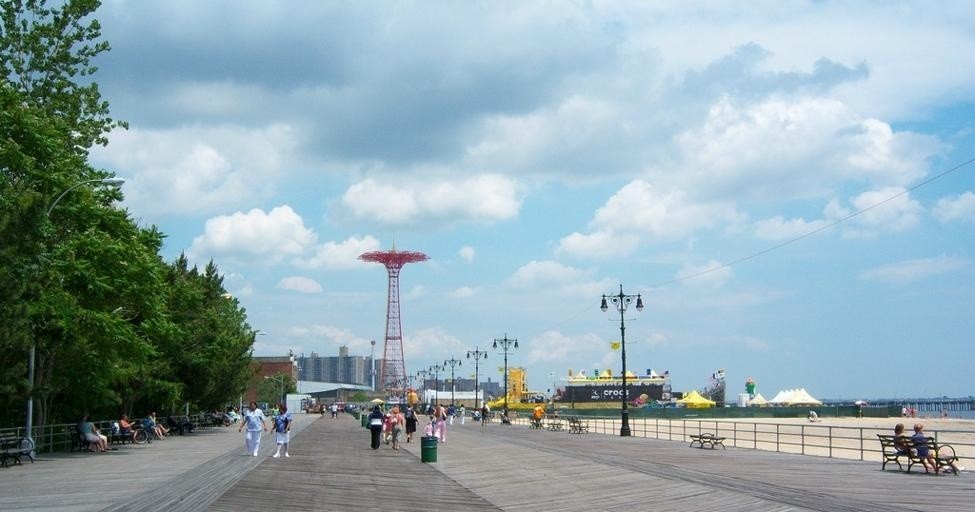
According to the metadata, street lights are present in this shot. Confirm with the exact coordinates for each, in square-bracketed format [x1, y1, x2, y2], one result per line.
[264, 374, 283, 404]
[428, 362, 444, 406]
[384, 369, 432, 414]
[19, 177, 125, 460]
[600, 283, 643, 436]
[443, 355, 461, 406]
[466, 344, 488, 420]
[289, 349, 305, 393]
[492, 333, 518, 425]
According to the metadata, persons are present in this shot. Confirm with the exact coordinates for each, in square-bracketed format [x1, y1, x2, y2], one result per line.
[424, 403, 491, 444]
[167, 407, 240, 436]
[910, 423, 944, 473]
[79, 412, 170, 453]
[500, 404, 545, 429]
[902, 405, 917, 417]
[366, 404, 419, 450]
[270, 403, 292, 458]
[237, 402, 268, 457]
[893, 423, 916, 457]
[320, 402, 339, 419]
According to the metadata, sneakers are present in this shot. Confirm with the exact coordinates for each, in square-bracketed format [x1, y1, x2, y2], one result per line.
[285, 452, 289, 458]
[273, 453, 280, 457]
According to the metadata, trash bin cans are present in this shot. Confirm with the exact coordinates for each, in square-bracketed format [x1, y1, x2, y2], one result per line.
[346, 408, 360, 420]
[362, 415, 367, 427]
[421, 436, 437, 462]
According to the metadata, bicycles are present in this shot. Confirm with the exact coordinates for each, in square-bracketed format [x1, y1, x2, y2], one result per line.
[132, 421, 156, 444]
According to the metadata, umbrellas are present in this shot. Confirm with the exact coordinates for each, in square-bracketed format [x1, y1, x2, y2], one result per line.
[854, 400, 867, 419]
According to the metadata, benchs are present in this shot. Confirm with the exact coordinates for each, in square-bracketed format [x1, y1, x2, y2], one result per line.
[877, 434, 960, 476]
[690, 432, 726, 449]
[0, 416, 222, 467]
[528, 415, 589, 434]
